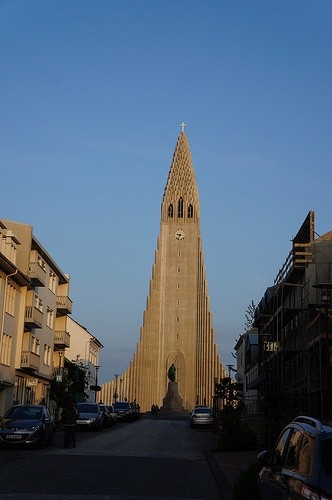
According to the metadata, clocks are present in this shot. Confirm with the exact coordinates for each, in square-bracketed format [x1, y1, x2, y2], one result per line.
[174, 229, 186, 241]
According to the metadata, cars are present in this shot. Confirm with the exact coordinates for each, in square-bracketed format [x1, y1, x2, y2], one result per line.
[0, 404, 55, 451]
[189, 405, 214, 429]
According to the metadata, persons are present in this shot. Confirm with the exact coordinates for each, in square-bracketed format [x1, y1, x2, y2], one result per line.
[61, 398, 79, 448]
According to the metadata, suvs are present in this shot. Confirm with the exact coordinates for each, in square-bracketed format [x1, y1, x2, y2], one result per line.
[75, 402, 140, 431]
[257, 416, 332, 500]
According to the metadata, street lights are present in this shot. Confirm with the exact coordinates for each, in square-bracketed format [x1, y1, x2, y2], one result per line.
[113, 374, 124, 404]
[225, 364, 234, 378]
[94, 365, 101, 403]
[54, 348, 66, 405]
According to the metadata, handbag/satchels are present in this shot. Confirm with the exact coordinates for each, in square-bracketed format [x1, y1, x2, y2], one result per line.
[73, 406, 79, 419]
